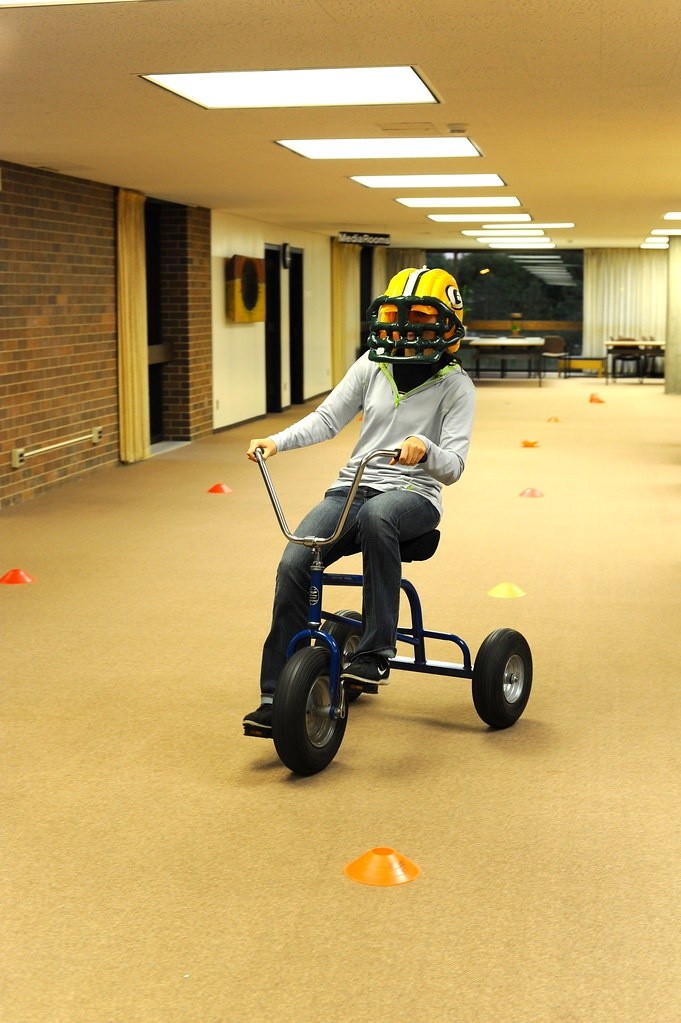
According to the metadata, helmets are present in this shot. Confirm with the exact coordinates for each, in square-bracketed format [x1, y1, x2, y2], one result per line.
[377, 265, 464, 357]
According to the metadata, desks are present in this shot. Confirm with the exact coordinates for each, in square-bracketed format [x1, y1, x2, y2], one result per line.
[604, 340, 668, 385]
[558, 355, 607, 376]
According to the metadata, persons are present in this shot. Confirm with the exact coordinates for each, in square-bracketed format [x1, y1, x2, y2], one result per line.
[242, 267, 478, 730]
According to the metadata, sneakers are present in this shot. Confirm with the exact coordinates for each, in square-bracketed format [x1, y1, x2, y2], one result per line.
[241, 703, 273, 730]
[338, 654, 391, 686]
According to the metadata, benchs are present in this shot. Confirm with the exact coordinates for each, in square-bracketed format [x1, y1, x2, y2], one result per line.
[471, 337, 545, 387]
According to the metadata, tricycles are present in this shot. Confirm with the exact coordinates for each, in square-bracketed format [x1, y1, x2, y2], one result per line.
[250, 446, 533, 774]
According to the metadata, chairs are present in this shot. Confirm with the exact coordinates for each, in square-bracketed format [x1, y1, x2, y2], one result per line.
[535, 334, 569, 378]
[613, 338, 666, 379]
